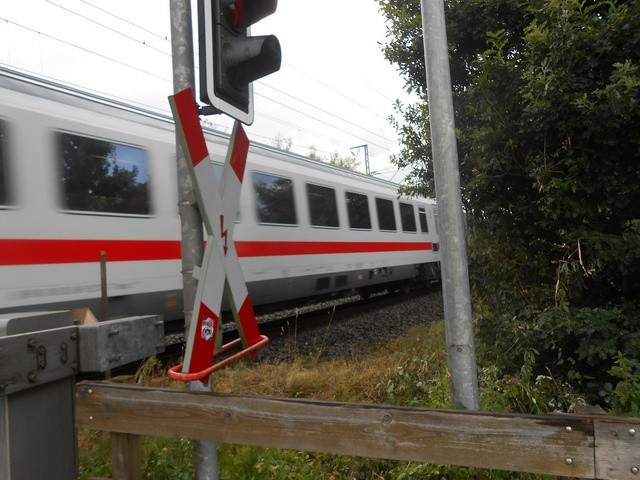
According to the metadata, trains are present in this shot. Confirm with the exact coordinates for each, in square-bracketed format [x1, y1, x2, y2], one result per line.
[0, 66, 489, 322]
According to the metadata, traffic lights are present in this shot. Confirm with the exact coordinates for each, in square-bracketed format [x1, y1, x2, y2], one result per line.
[197, 0, 281, 126]
